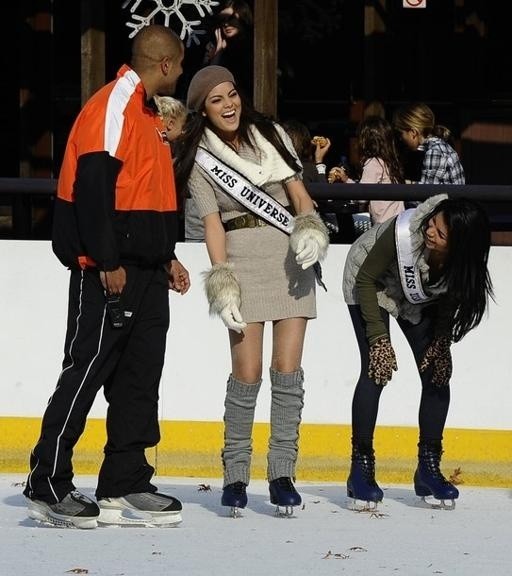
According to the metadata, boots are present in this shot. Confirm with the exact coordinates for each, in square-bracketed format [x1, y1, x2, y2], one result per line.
[26, 488, 181, 520]
[347, 448, 383, 501]
[221, 482, 248, 509]
[414, 452, 459, 499]
[269, 478, 301, 506]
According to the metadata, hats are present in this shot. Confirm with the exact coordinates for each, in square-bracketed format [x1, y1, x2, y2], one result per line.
[186, 65, 236, 113]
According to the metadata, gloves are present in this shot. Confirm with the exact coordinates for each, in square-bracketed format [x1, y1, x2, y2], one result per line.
[368, 333, 398, 386]
[419, 335, 452, 387]
[289, 213, 329, 270]
[201, 265, 246, 333]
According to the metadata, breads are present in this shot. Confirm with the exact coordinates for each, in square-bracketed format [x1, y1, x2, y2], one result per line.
[328, 170, 338, 184]
[310, 136, 327, 147]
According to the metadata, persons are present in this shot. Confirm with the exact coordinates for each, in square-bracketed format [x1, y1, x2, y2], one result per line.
[201, 1, 252, 64]
[174, 64, 332, 509]
[392, 98, 466, 185]
[334, 113, 405, 225]
[281, 117, 332, 213]
[21, 24, 191, 518]
[158, 95, 190, 144]
[342, 193, 495, 502]
